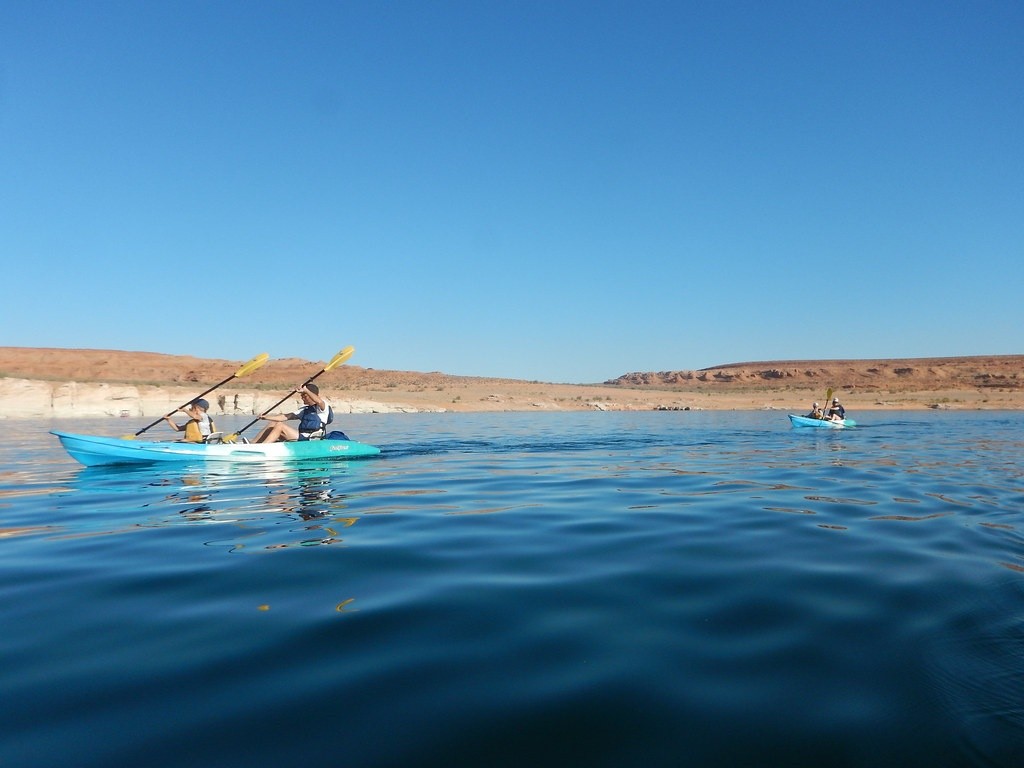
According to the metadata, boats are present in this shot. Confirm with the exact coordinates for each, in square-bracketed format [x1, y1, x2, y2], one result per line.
[788, 412, 857, 429]
[49, 426, 383, 469]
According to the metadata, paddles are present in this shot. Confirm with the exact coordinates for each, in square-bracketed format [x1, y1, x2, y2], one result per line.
[222, 344, 356, 442]
[823, 387, 833, 417]
[120, 352, 270, 439]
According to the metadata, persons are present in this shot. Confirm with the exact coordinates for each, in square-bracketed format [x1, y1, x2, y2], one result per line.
[243, 384, 330, 445]
[802, 402, 823, 420]
[163, 398, 215, 444]
[825, 398, 846, 421]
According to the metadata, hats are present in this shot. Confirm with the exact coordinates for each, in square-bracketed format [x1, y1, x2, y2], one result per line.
[813, 403, 819, 407]
[191, 399, 209, 410]
[833, 398, 838, 402]
[306, 384, 321, 396]
